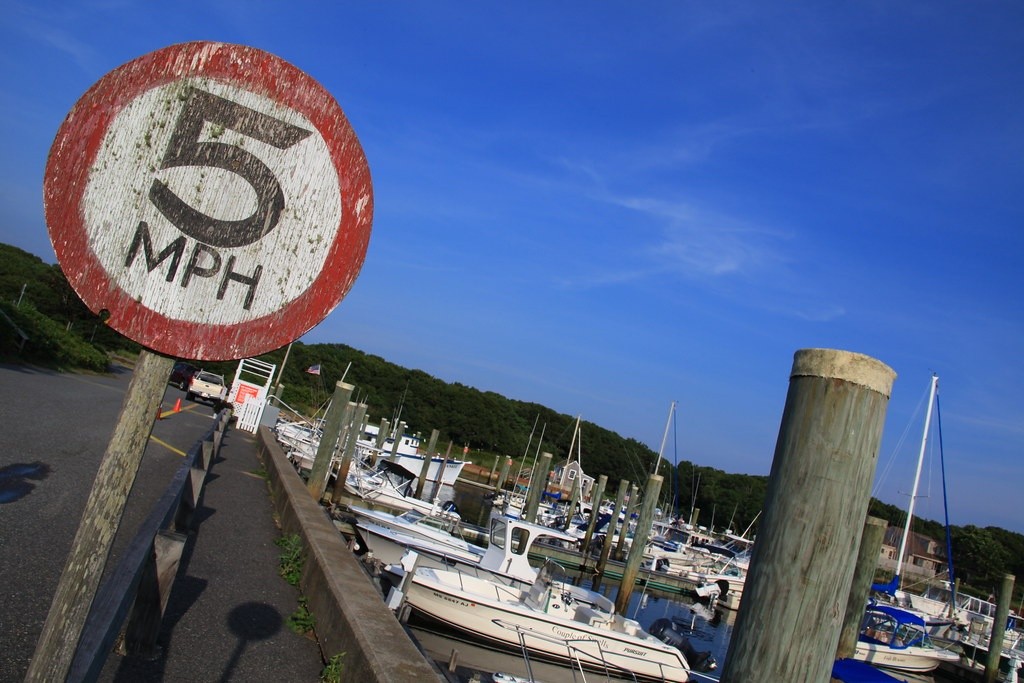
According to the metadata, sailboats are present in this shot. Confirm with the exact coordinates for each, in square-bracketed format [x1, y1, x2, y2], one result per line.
[852, 371, 1024, 675]
[273, 360, 764, 612]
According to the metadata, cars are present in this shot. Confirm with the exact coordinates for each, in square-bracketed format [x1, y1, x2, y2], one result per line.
[170, 362, 200, 391]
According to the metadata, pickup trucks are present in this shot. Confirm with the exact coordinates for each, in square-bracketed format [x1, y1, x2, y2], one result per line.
[187, 369, 228, 407]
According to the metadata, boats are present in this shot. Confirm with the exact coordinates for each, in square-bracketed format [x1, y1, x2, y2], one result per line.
[379, 548, 694, 683]
[346, 503, 549, 580]
[354, 515, 616, 619]
